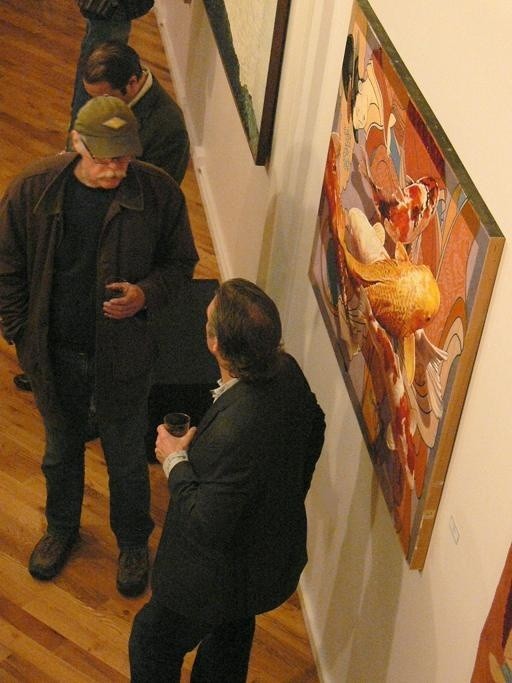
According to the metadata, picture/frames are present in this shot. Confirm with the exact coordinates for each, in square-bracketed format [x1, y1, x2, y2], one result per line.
[202, 0, 290, 164]
[306, 1, 500, 572]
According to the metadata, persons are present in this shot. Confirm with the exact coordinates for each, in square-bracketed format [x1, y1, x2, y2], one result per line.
[15, 39, 190, 446]
[66, 0, 154, 150]
[0, 94, 200, 601]
[127, 277, 328, 683]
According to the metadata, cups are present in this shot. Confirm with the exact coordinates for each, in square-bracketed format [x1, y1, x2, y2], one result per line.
[104, 276, 129, 301]
[164, 412, 192, 437]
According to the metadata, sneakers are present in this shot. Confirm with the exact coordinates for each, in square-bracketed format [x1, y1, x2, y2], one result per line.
[27, 524, 78, 580]
[116, 539, 150, 598]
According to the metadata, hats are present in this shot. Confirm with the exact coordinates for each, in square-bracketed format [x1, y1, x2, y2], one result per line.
[74, 94, 144, 159]
